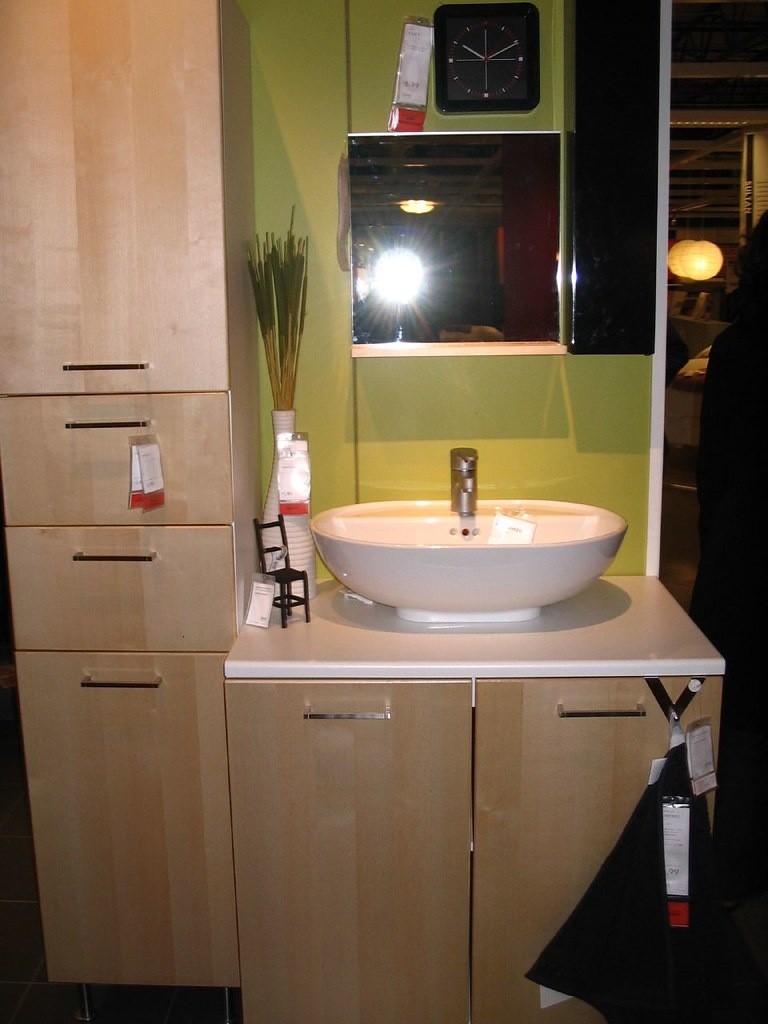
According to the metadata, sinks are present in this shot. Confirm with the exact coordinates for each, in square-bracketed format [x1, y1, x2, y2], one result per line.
[309, 498, 630, 625]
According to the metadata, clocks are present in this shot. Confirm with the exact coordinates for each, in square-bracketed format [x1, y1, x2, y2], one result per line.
[430, 2, 539, 114]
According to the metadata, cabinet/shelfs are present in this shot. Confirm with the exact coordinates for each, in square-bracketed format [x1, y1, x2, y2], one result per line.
[0, 0, 723, 1024]
[665, 309, 730, 490]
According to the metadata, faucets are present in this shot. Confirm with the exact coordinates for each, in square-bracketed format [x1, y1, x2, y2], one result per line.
[450, 448, 479, 519]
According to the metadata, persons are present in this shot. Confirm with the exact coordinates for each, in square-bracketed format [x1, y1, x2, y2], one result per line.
[690, 209, 768, 908]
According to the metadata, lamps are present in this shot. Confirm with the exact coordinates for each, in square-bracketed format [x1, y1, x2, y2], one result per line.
[668, 156, 723, 280]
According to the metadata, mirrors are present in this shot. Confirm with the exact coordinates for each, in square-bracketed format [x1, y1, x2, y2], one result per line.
[348, 131, 569, 358]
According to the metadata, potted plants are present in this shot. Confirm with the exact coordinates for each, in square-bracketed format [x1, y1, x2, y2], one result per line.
[244, 202, 318, 603]
